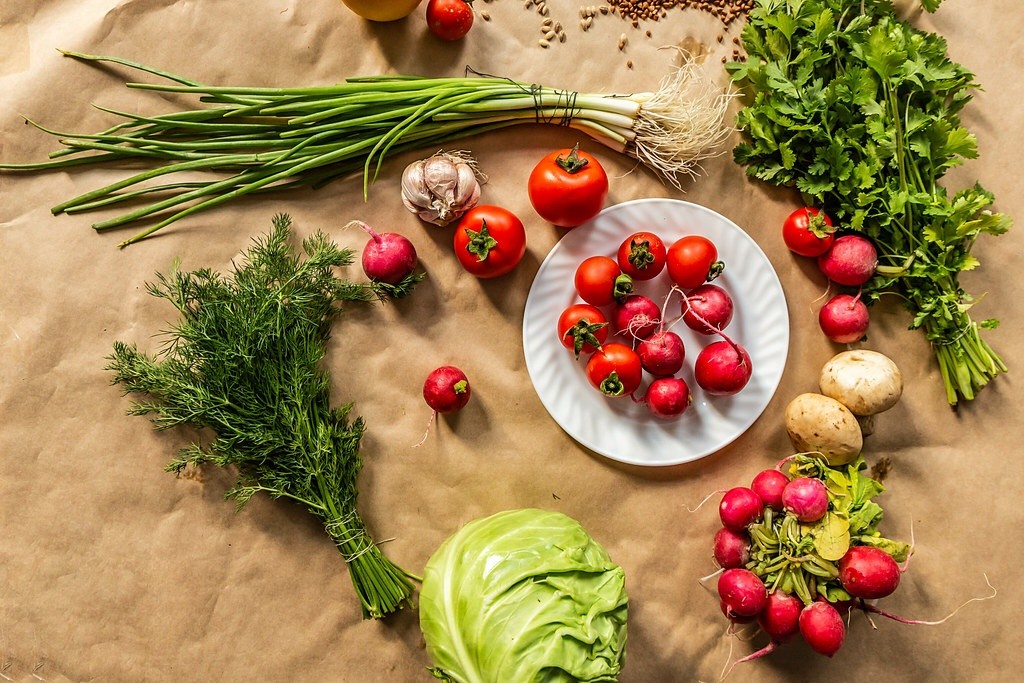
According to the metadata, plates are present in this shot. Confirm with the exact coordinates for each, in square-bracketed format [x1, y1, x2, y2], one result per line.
[522, 198, 790, 467]
